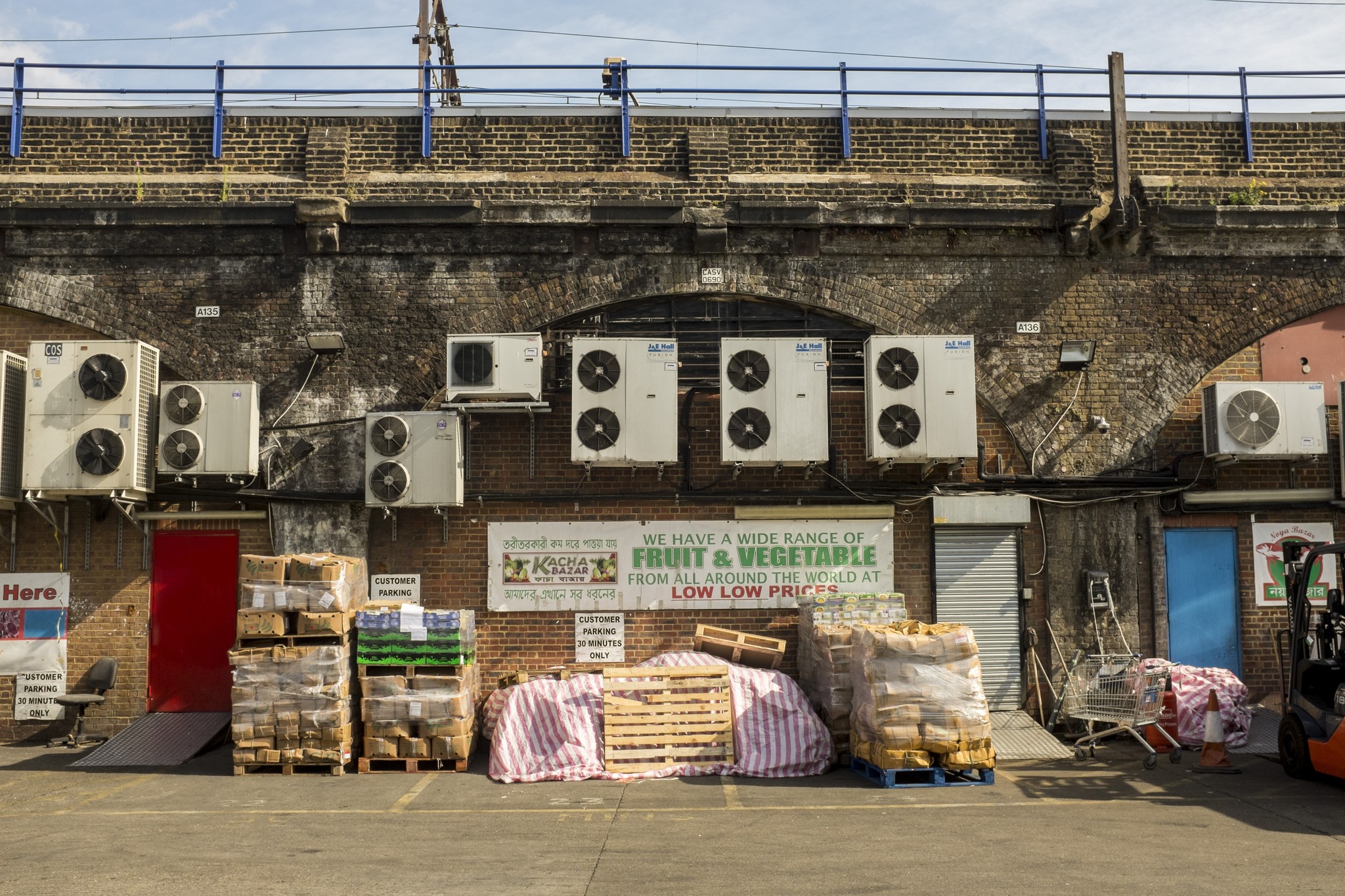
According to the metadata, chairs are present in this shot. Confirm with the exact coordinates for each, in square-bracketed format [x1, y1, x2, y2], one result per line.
[48, 657, 118, 748]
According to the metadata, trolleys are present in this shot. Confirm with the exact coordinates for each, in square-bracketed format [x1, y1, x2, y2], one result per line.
[1062, 653, 1183, 769]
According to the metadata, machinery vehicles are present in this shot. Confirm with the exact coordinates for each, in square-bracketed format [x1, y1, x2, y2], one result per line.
[1277, 541, 1345, 785]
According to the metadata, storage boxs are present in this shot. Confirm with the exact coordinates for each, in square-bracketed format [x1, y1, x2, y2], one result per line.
[797, 594, 995, 770]
[227, 554, 482, 764]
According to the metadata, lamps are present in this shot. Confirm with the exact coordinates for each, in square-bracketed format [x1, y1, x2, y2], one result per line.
[133, 510, 267, 520]
[1183, 488, 1336, 503]
[306, 331, 346, 355]
[1059, 339, 1097, 371]
[735, 504, 896, 519]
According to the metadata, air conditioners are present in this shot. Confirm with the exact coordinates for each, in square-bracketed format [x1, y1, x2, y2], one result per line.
[0, 350, 28, 512]
[864, 335, 978, 463]
[446, 332, 544, 402]
[157, 382, 258, 476]
[1203, 381, 1329, 459]
[571, 337, 679, 468]
[365, 412, 464, 506]
[719, 338, 828, 466]
[20, 339, 159, 496]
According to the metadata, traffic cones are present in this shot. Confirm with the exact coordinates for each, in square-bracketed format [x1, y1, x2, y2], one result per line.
[1191, 689, 1247, 774]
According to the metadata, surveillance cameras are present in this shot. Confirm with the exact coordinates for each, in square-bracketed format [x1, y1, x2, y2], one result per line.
[1096, 422, 1110, 435]
